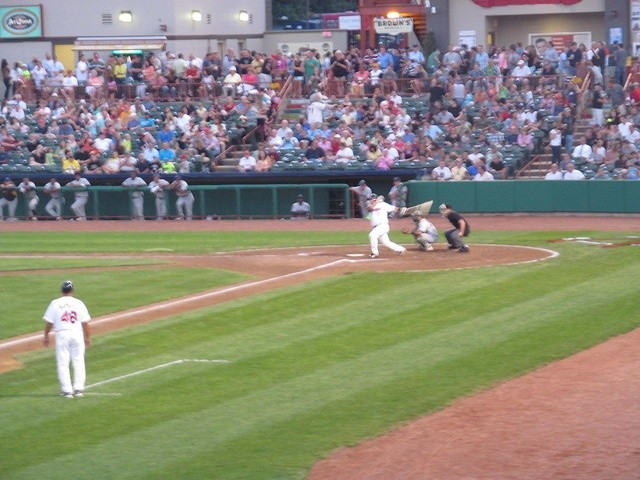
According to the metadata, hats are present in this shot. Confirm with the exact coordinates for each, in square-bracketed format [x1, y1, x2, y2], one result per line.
[357, 178, 366, 186]
[174, 174, 183, 180]
[366, 194, 377, 200]
[62, 281, 74, 290]
[438, 203, 451, 209]
[392, 177, 401, 182]
[296, 194, 304, 199]
[411, 210, 423, 216]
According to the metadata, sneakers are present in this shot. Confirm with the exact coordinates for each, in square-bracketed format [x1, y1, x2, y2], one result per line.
[459, 246, 469, 251]
[370, 253, 377, 258]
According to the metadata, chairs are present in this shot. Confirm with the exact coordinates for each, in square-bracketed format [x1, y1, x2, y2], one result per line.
[558, 64, 639, 180]
[0, 81, 252, 174]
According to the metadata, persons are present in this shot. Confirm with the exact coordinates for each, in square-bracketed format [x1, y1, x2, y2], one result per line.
[146, 172, 171, 220]
[168, 174, 196, 220]
[0, 177, 18, 221]
[121, 170, 148, 220]
[18, 177, 39, 221]
[42, 280, 92, 399]
[290, 193, 310, 219]
[348, 179, 374, 218]
[401, 210, 439, 252]
[366, 194, 410, 259]
[276, 39, 640, 180]
[42, 177, 62, 221]
[438, 203, 471, 252]
[65, 171, 91, 221]
[0, 48, 276, 171]
[387, 176, 408, 217]
